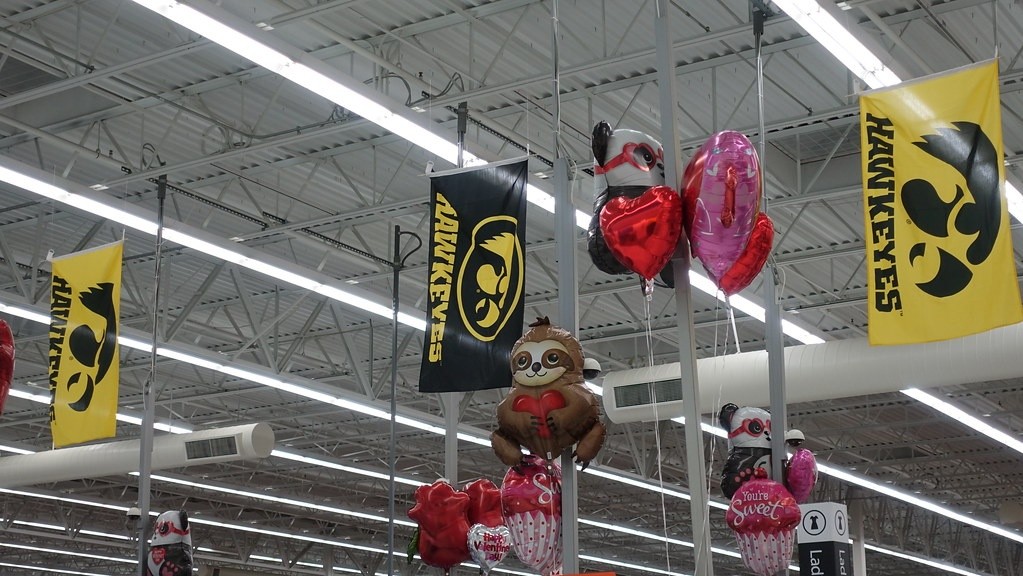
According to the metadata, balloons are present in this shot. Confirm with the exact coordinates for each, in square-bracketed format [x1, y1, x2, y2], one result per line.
[725, 478, 802, 576]
[680, 130, 762, 281]
[785, 447, 818, 503]
[710, 212, 775, 295]
[490, 318, 606, 474]
[146, 510, 193, 576]
[0, 319, 15, 417]
[405, 454, 563, 576]
[719, 404, 773, 499]
[585, 119, 683, 288]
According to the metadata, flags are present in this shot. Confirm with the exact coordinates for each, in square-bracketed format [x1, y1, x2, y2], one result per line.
[419, 160, 525, 393]
[858, 62, 1023, 347]
[49, 241, 120, 448]
